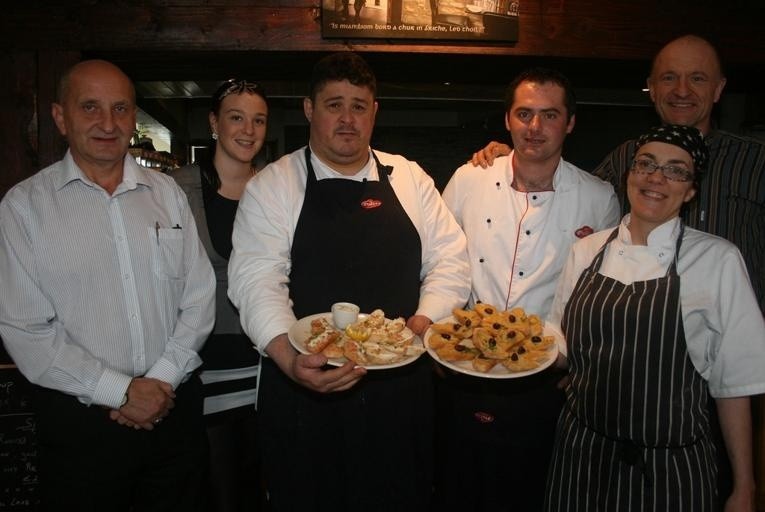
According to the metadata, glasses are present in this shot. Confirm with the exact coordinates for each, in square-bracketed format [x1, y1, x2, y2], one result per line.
[628, 158, 696, 181]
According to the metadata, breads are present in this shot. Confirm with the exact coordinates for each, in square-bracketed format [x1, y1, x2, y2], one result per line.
[304, 309, 426, 366]
[428, 304, 555, 373]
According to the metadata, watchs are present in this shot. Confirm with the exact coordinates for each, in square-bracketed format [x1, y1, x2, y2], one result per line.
[120, 392, 128, 407]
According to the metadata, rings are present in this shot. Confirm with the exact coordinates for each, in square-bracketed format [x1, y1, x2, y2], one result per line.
[155, 419, 161, 424]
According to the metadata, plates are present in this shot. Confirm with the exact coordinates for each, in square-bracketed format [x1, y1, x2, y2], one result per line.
[287, 312, 560, 378]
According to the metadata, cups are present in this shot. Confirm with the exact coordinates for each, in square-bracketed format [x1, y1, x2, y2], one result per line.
[333, 300, 360, 328]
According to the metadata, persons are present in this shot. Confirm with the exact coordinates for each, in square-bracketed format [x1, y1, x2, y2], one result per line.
[540, 123, 764, 509]
[168, 52, 619, 511]
[1, 58, 217, 512]
[472, 36, 764, 313]
